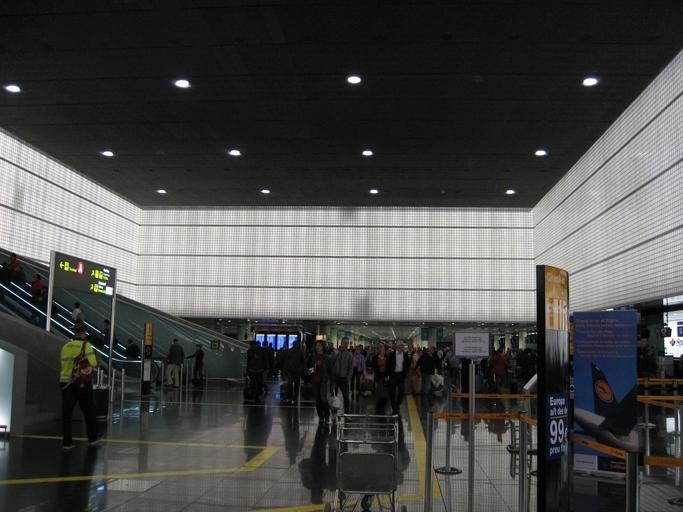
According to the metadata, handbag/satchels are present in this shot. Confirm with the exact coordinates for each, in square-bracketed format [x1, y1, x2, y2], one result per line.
[431, 372, 445, 388]
[409, 369, 424, 396]
[309, 371, 321, 382]
[72, 340, 93, 387]
[359, 370, 376, 398]
[326, 387, 344, 411]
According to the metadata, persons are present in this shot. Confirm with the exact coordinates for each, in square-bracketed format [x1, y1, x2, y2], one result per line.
[180, 390, 203, 431]
[55, 446, 99, 511]
[184, 343, 205, 390]
[0, 252, 141, 360]
[58, 321, 101, 451]
[241, 402, 537, 504]
[243, 335, 534, 427]
[164, 339, 185, 388]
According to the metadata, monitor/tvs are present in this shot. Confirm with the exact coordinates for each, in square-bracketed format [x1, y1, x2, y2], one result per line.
[267, 334, 276, 349]
[287, 334, 298, 349]
[277, 334, 286, 351]
[256, 333, 265, 346]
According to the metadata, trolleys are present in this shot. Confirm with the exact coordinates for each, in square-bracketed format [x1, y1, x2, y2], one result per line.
[324, 411, 407, 512]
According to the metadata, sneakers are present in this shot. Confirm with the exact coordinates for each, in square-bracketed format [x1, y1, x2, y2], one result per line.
[62, 438, 100, 450]
